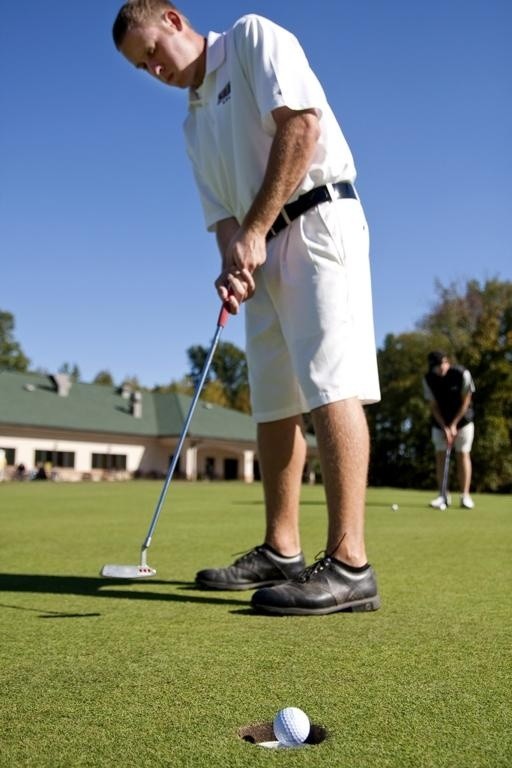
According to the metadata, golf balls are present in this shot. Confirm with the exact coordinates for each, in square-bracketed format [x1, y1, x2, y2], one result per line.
[273, 708, 312, 746]
[440, 504, 447, 511]
[392, 504, 398, 510]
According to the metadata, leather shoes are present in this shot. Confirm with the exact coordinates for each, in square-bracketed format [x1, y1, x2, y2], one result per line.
[248, 551, 387, 618]
[190, 541, 310, 593]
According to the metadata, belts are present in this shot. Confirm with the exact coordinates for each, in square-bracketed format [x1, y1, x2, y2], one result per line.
[252, 177, 360, 243]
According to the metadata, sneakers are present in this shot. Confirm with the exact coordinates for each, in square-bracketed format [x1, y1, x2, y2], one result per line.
[429, 494, 452, 509]
[459, 494, 475, 509]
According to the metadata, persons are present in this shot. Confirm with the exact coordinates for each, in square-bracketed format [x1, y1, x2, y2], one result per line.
[111, 0, 382, 615]
[422, 350, 475, 510]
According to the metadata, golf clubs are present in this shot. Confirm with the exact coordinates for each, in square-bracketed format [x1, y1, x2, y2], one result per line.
[101, 307, 230, 579]
[442, 436, 454, 506]
